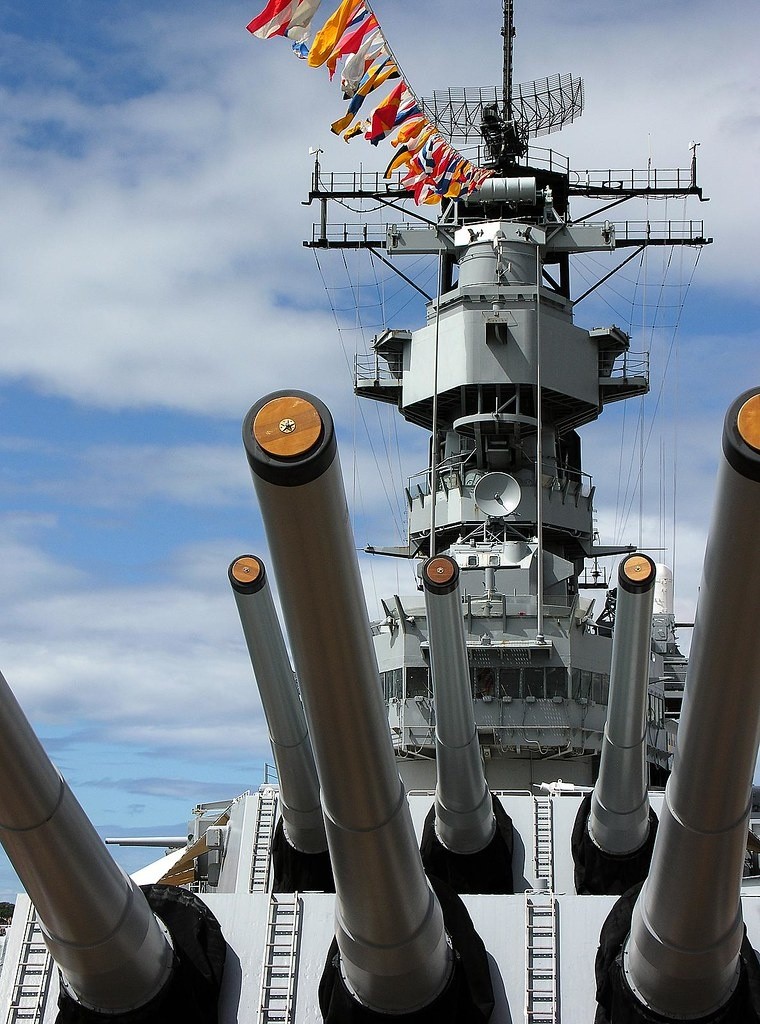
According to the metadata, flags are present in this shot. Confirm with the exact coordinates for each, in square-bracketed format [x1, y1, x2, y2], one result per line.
[365, 81, 405, 140]
[245, 0, 495, 204]
